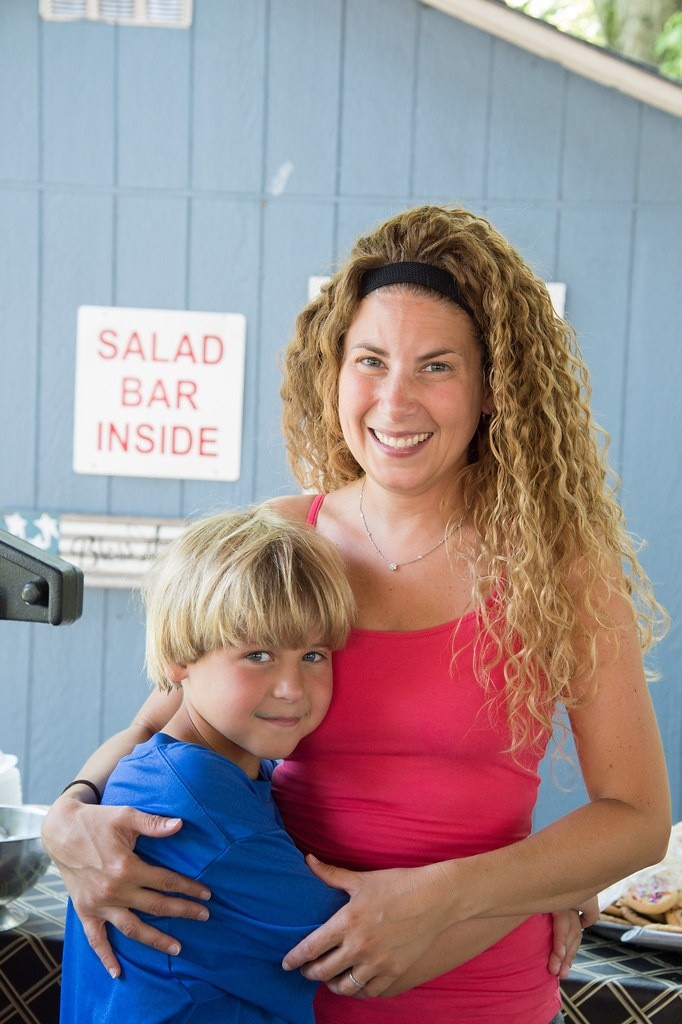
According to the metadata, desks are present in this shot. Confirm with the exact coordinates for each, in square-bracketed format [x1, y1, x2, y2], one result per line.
[1, 871, 682, 1024]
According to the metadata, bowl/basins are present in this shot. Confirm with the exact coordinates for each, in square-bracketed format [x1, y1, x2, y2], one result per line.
[0, 803, 52, 932]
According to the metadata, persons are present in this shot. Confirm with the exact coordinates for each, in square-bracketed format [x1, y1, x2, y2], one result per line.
[58, 511, 598, 1024]
[42, 208, 671, 1024]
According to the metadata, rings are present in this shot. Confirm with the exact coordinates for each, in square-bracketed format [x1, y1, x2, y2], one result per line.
[349, 969, 366, 988]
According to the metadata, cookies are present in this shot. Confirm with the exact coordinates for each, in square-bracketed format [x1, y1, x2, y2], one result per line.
[597, 885, 682, 933]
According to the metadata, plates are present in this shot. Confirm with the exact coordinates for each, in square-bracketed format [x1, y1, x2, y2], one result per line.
[584, 920, 682, 949]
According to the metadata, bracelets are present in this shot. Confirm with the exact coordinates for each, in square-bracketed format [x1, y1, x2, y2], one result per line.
[61, 780, 101, 804]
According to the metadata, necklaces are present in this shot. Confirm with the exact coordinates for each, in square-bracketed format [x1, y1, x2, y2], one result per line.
[360, 476, 476, 571]
